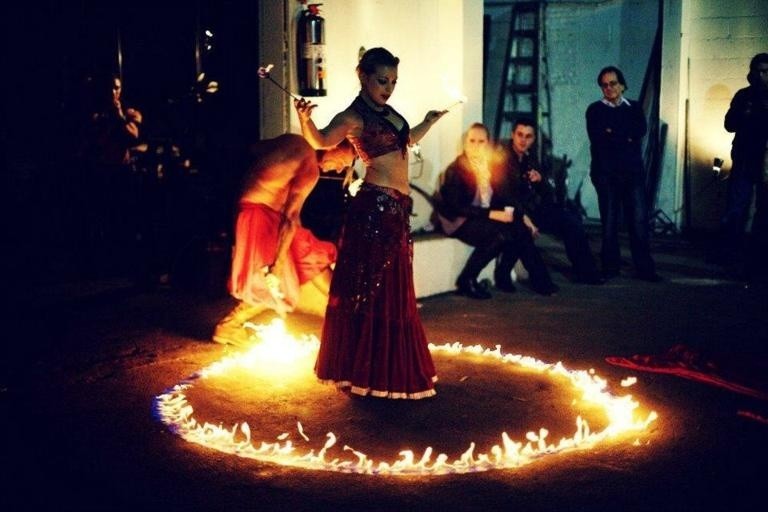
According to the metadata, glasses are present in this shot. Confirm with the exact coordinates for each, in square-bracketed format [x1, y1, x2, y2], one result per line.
[599, 79, 619, 88]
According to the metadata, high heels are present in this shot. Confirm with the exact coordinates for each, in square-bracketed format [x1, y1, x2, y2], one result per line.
[454, 279, 491, 298]
[494, 269, 517, 294]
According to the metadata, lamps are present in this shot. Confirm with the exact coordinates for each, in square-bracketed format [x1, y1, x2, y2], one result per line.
[712, 158, 723, 174]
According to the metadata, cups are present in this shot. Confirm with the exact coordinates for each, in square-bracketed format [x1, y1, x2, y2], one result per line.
[505, 206, 514, 214]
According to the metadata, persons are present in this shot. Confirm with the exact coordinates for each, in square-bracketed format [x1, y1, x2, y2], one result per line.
[212, 134, 357, 350]
[586, 66, 655, 280]
[724, 53, 768, 244]
[496, 119, 585, 300]
[428, 124, 520, 298]
[296, 48, 442, 401]
[89, 78, 144, 180]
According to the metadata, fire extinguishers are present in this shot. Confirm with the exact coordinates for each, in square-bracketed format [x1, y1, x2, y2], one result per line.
[295, 2, 330, 98]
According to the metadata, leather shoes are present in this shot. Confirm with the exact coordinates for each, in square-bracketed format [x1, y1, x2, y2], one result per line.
[213, 325, 257, 348]
